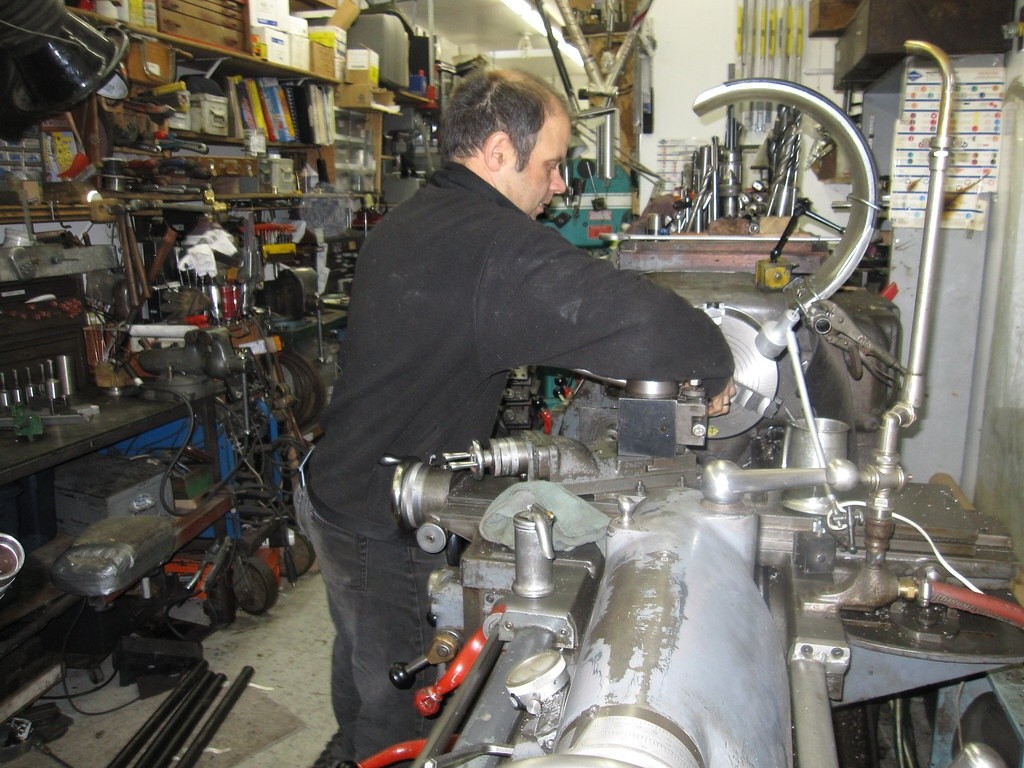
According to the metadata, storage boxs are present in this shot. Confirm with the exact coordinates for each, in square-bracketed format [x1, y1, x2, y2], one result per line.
[158, 0, 410, 195]
[807, 0, 1016, 92]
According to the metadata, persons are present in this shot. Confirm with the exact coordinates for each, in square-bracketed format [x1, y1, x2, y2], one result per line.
[293, 67, 736, 768]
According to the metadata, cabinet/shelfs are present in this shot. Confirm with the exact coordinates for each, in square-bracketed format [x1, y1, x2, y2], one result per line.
[96, 302, 348, 539]
[1, 273, 90, 395]
[0, 363, 232, 638]
[0, 18, 390, 308]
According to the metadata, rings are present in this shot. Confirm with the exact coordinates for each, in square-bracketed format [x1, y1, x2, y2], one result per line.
[723, 402, 731, 405]
